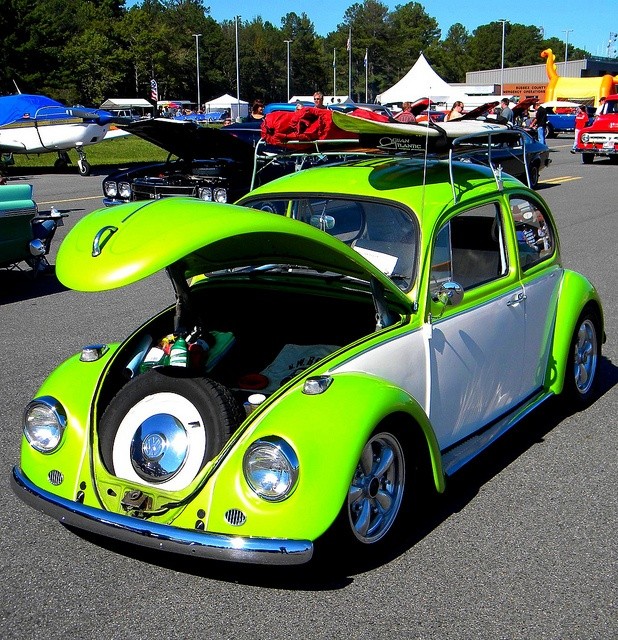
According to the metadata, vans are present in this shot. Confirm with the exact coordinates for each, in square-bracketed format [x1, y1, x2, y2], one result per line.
[330, 103, 393, 118]
[262, 102, 342, 120]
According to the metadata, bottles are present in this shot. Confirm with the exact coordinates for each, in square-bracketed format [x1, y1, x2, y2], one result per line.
[140, 337, 170, 374]
[50, 205, 64, 227]
[169, 334, 190, 368]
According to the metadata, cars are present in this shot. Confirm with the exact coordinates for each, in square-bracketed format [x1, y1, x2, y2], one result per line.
[102, 119, 363, 210]
[541, 102, 596, 138]
[571, 94, 618, 164]
[9, 153, 607, 571]
[394, 127, 552, 188]
[1, 181, 70, 283]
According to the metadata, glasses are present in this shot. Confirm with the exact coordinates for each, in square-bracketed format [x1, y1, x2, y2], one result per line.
[314, 98, 321, 100]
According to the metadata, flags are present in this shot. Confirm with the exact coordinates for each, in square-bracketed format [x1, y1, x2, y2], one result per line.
[151, 79, 157, 101]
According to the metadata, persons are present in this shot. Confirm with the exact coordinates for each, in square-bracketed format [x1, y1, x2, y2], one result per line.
[443, 101, 470, 122]
[313, 92, 326, 110]
[394, 102, 417, 124]
[529, 103, 547, 145]
[594, 97, 608, 119]
[245, 99, 266, 122]
[494, 98, 512, 125]
[569, 105, 589, 154]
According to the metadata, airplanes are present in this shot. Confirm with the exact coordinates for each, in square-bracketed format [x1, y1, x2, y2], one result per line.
[1, 78, 189, 176]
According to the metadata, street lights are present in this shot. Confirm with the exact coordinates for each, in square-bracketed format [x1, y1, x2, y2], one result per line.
[192, 33, 203, 114]
[562, 29, 573, 77]
[283, 40, 294, 103]
[497, 19, 510, 95]
